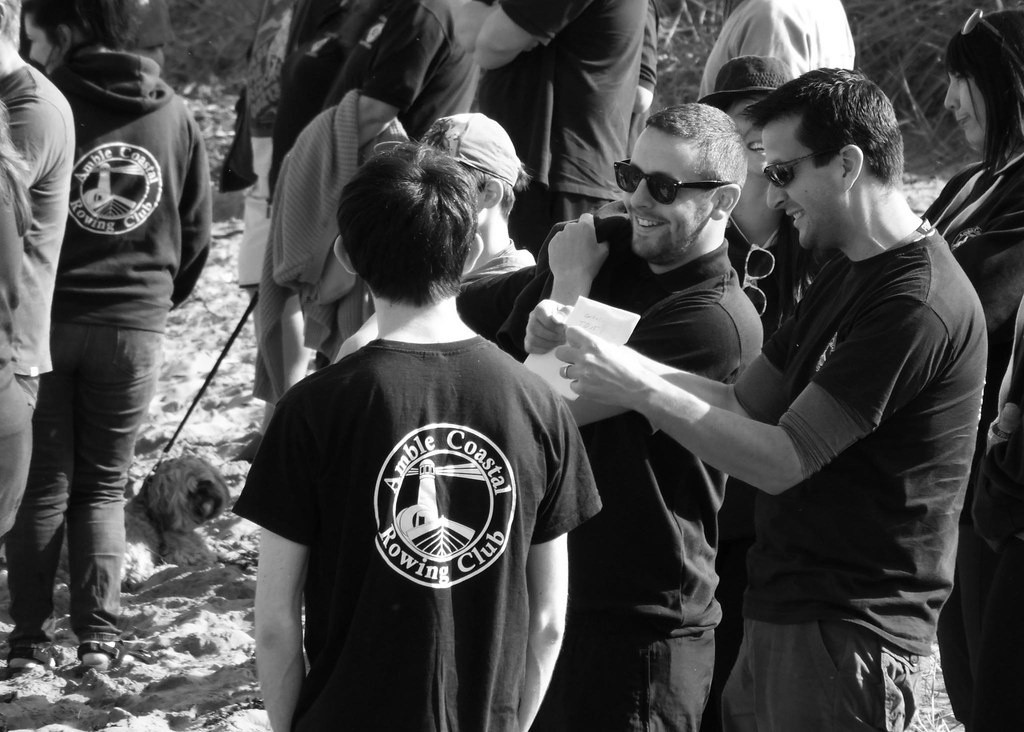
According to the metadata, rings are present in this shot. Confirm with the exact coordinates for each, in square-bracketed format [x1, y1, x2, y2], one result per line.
[564, 364, 571, 378]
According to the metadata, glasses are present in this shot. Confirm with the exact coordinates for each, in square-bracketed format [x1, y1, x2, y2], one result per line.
[741, 241, 775, 317]
[613, 159, 732, 205]
[764, 143, 856, 188]
[960, 8, 1002, 36]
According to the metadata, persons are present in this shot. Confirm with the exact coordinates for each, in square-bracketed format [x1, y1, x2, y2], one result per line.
[0, 3, 75, 536]
[7, 0, 210, 676]
[918, 9, 1024, 732]
[523, 56, 989, 732]
[457, 103, 763, 732]
[252, 0, 659, 408]
[234, 144, 602, 732]
[697, 0, 856, 104]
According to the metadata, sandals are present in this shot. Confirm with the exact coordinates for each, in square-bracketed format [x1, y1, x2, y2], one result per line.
[77, 639, 161, 673]
[7, 647, 63, 674]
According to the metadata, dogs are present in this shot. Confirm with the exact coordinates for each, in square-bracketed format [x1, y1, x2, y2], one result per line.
[55, 453, 250, 595]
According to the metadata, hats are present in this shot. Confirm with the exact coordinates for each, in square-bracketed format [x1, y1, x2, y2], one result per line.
[696, 55, 794, 112]
[373, 112, 521, 187]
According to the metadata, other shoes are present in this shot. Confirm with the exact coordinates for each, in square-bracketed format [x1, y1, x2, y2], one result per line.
[228, 433, 262, 464]
